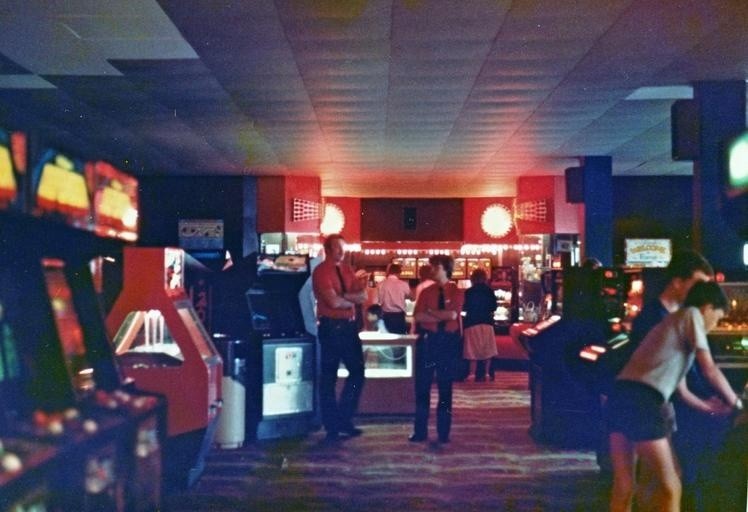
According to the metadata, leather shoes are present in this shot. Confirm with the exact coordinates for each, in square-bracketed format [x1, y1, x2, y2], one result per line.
[324, 423, 362, 443]
[409, 434, 448, 443]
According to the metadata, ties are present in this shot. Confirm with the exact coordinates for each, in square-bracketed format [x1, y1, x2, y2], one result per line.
[336, 265, 347, 293]
[438, 287, 445, 334]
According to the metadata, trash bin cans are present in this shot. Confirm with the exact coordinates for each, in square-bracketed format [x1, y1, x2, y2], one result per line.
[214, 375, 247, 450]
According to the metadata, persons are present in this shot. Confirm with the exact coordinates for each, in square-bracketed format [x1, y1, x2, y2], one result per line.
[414, 266, 434, 302]
[377, 264, 411, 333]
[629, 247, 720, 425]
[461, 269, 498, 381]
[366, 304, 395, 365]
[606, 279, 743, 512]
[405, 254, 460, 445]
[354, 269, 376, 330]
[312, 234, 365, 444]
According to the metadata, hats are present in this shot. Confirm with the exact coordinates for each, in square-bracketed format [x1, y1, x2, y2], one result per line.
[356, 269, 372, 280]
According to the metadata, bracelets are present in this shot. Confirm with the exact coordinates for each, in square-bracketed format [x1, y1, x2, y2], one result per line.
[732, 398, 743, 410]
[427, 306, 432, 314]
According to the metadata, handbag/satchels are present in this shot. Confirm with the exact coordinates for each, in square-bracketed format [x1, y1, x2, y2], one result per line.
[523, 301, 538, 322]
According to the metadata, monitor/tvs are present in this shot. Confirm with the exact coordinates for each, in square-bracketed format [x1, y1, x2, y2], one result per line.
[39, 260, 98, 375]
[175, 302, 218, 365]
[245, 289, 305, 333]
